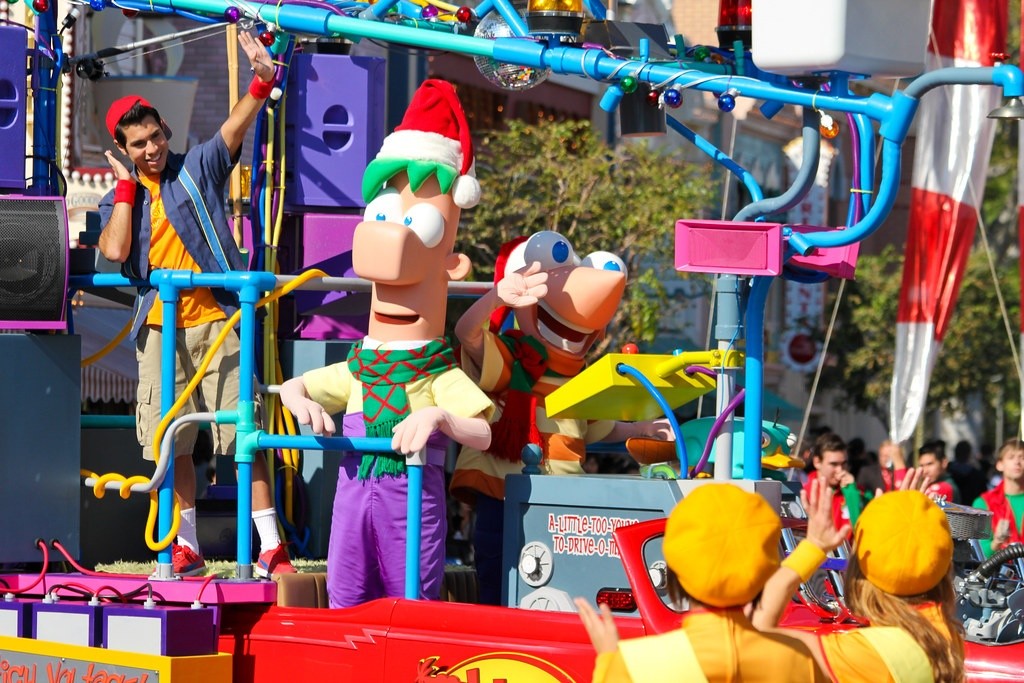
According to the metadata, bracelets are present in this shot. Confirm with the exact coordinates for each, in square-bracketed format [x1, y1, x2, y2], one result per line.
[780, 540, 828, 583]
[249, 74, 276, 100]
[113, 180, 136, 207]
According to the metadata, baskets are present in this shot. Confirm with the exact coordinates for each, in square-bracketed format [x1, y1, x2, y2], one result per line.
[939, 499, 994, 539]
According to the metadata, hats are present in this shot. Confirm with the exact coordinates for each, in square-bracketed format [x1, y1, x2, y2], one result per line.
[661, 482, 781, 607]
[106, 96, 172, 141]
[850, 490, 953, 595]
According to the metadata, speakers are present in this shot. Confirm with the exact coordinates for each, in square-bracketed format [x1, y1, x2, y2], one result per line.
[276, 214, 373, 340]
[752, 0, 935, 79]
[0, 333, 81, 559]
[281, 51, 386, 208]
[0, 194, 69, 328]
[0, 25, 28, 188]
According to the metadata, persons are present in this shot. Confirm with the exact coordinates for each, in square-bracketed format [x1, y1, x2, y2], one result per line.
[879, 439, 960, 504]
[972, 439, 1024, 562]
[804, 425, 995, 507]
[743, 467, 966, 683]
[449, 230, 670, 606]
[97, 30, 297, 580]
[573, 481, 827, 683]
[280, 79, 496, 609]
[803, 433, 873, 548]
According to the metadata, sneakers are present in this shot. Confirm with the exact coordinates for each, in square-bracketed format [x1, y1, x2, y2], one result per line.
[152, 542, 206, 578]
[255, 541, 297, 578]
